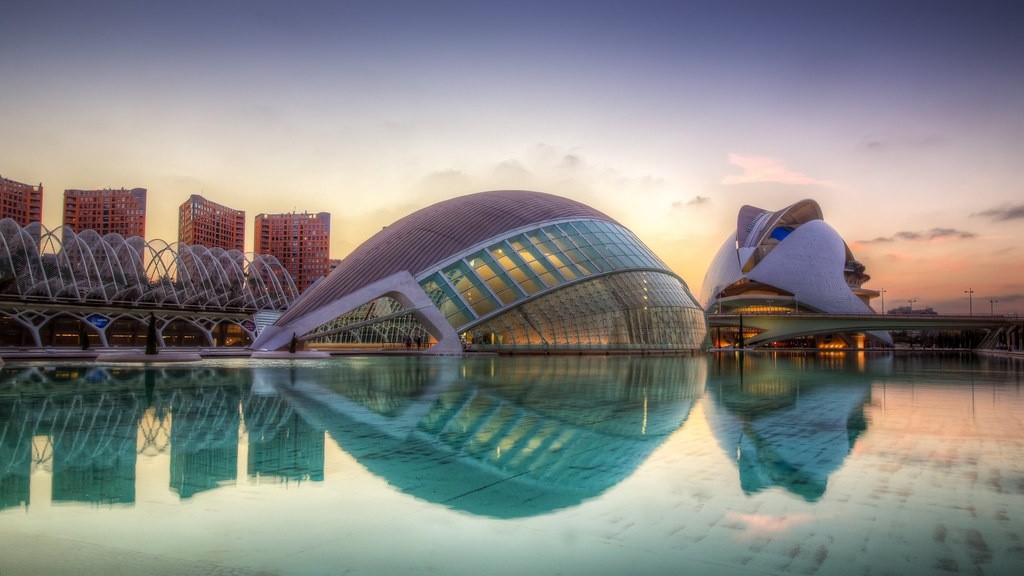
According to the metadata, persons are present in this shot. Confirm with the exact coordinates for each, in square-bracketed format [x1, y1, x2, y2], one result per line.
[405, 334, 422, 350]
